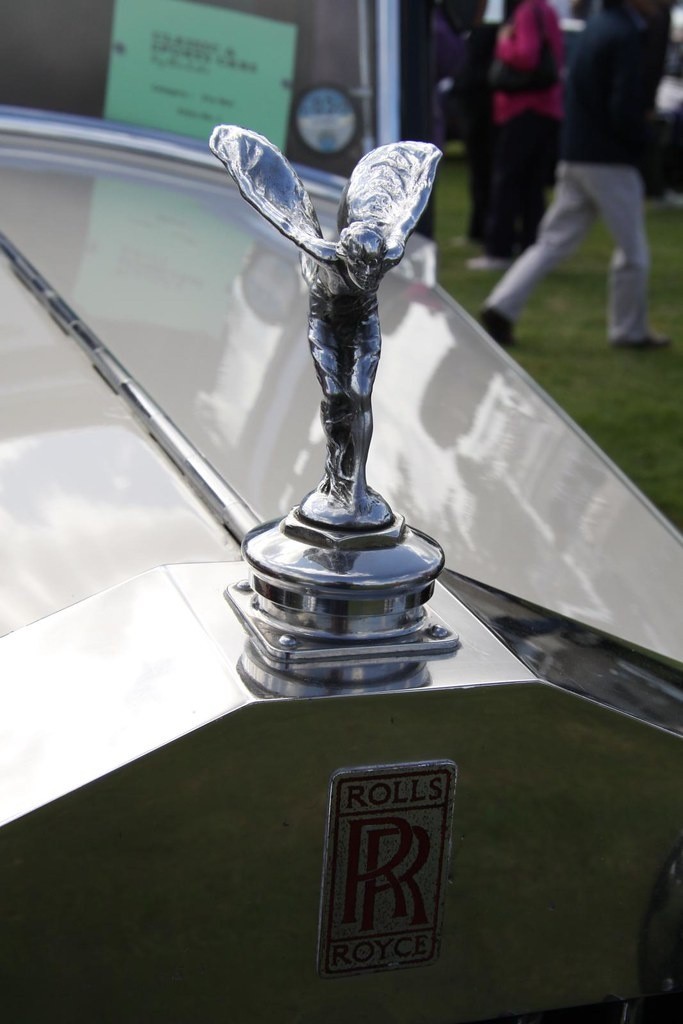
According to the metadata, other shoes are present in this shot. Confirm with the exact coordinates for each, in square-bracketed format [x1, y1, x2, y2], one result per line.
[482, 310, 520, 346]
[608, 331, 674, 351]
[465, 255, 516, 271]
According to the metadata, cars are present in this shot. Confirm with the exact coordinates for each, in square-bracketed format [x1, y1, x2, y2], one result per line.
[0, 0, 683, 1024]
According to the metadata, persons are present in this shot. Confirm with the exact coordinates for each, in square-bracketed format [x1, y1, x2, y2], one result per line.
[465, 1, 564, 271]
[473, 0, 674, 353]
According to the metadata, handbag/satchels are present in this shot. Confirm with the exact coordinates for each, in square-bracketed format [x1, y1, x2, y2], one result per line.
[489, 1, 556, 93]
